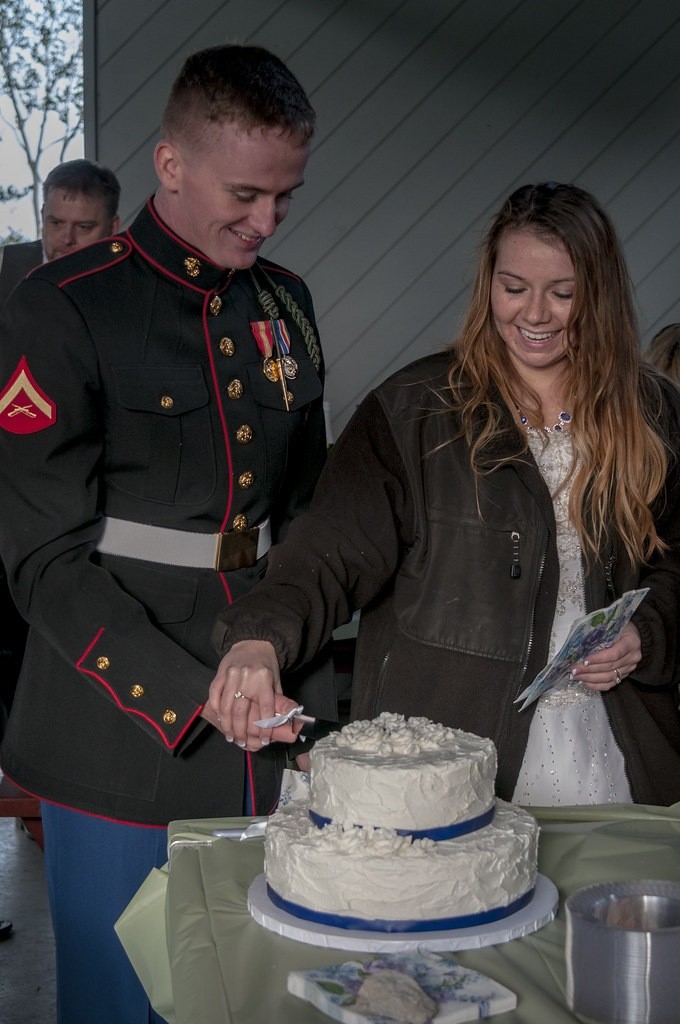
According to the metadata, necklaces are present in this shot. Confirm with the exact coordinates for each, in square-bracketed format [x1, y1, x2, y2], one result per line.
[514, 404, 572, 433]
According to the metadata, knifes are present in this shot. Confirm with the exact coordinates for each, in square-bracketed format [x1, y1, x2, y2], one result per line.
[267, 713, 352, 744]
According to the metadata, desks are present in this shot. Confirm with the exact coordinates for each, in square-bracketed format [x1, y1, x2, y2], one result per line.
[108, 798, 680, 1024]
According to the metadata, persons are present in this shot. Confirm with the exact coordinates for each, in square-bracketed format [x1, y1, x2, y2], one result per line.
[209, 181, 680, 805]
[0, 159, 121, 310]
[0, 43, 337, 1024]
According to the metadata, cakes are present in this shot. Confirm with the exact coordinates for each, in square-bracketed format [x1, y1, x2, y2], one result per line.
[263, 711, 542, 931]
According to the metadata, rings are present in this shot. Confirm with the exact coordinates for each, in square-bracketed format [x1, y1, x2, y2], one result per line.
[614, 677, 621, 684]
[234, 691, 250, 701]
[614, 669, 622, 679]
